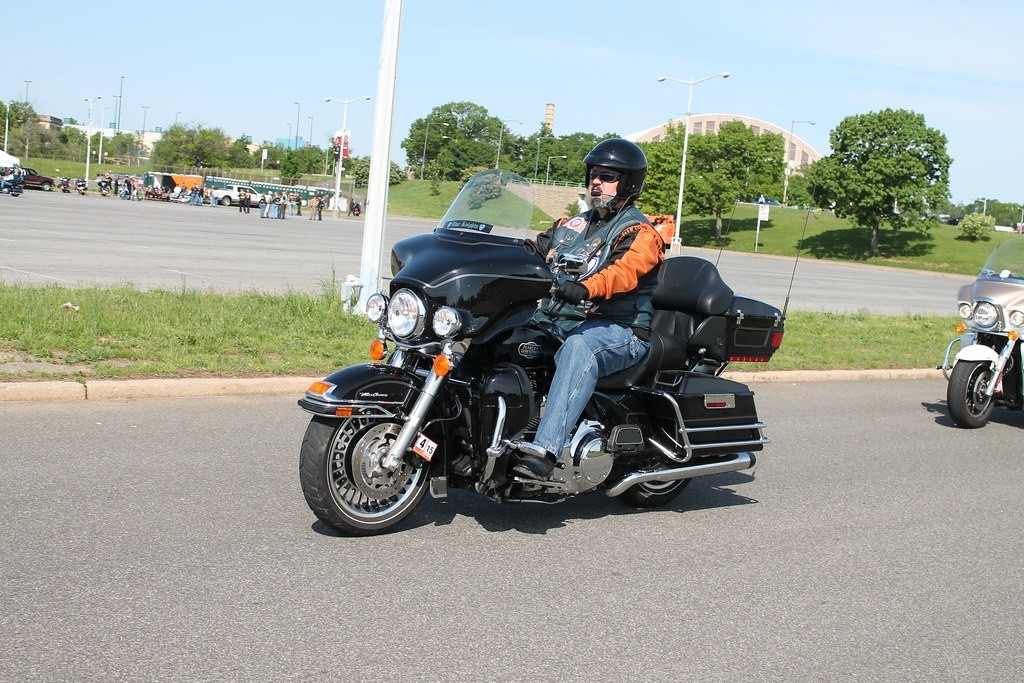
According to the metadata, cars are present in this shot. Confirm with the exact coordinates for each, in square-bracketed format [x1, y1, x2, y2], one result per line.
[751, 196, 780, 206]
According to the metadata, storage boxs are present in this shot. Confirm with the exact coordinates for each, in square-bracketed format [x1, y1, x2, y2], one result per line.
[725, 295, 786, 363]
[649, 373, 768, 455]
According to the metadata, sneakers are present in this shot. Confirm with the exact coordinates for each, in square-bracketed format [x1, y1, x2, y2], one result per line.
[512, 449, 555, 481]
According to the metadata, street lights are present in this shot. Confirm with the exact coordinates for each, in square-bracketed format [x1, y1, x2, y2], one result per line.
[24, 80, 32, 103]
[82, 75, 183, 183]
[286, 101, 314, 151]
[658, 71, 731, 257]
[324, 96, 372, 218]
[535, 136, 568, 185]
[782, 120, 817, 205]
[420, 123, 450, 180]
[494, 120, 524, 168]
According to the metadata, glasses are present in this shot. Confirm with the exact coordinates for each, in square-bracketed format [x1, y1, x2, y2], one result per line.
[589, 168, 622, 183]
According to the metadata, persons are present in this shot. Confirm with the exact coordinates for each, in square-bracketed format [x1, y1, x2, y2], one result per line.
[518, 137, 664, 482]
[309, 195, 325, 220]
[97, 170, 140, 201]
[260, 191, 302, 219]
[145, 183, 215, 207]
[1, 164, 26, 189]
[348, 199, 355, 215]
[238, 189, 251, 213]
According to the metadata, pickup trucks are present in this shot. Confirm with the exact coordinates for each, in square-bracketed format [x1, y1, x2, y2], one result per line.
[208, 185, 268, 209]
[0, 168, 54, 191]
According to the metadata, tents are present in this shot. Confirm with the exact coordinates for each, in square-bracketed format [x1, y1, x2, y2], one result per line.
[0, 150, 19, 168]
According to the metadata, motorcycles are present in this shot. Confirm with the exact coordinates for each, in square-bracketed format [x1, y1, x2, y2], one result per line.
[0, 179, 26, 197]
[75, 178, 88, 195]
[934, 235, 1024, 429]
[295, 169, 816, 538]
[119, 185, 129, 199]
[353, 203, 362, 216]
[60, 177, 71, 193]
[98, 181, 109, 196]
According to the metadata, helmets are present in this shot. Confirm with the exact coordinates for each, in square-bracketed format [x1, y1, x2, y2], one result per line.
[583, 139, 647, 203]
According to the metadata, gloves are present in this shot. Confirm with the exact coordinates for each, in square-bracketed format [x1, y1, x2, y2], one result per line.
[555, 280, 588, 307]
